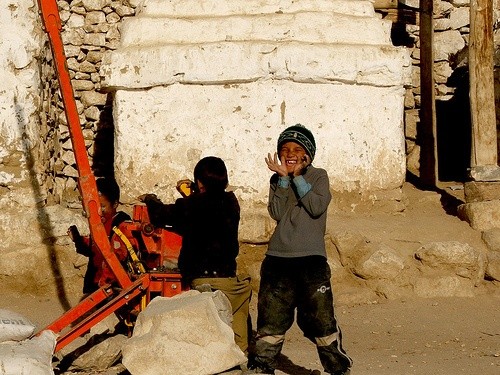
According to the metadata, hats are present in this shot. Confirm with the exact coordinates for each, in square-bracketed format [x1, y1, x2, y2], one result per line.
[277, 124, 316, 161]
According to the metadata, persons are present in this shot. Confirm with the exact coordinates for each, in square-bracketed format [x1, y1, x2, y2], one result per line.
[66, 177, 139, 341]
[249, 124, 353, 375]
[136, 155, 253, 373]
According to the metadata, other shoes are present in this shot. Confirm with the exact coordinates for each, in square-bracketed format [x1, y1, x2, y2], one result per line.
[240, 365, 274, 375]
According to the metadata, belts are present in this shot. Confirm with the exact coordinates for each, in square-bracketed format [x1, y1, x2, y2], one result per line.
[199, 274, 235, 278]
